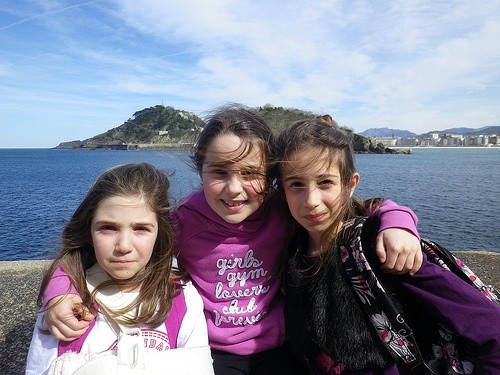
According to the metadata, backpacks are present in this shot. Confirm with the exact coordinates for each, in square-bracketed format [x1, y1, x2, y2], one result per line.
[338, 214, 500, 375]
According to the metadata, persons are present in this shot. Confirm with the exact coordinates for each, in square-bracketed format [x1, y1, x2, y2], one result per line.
[43, 103, 422, 375]
[24, 162, 213, 375]
[275, 117, 500, 375]
